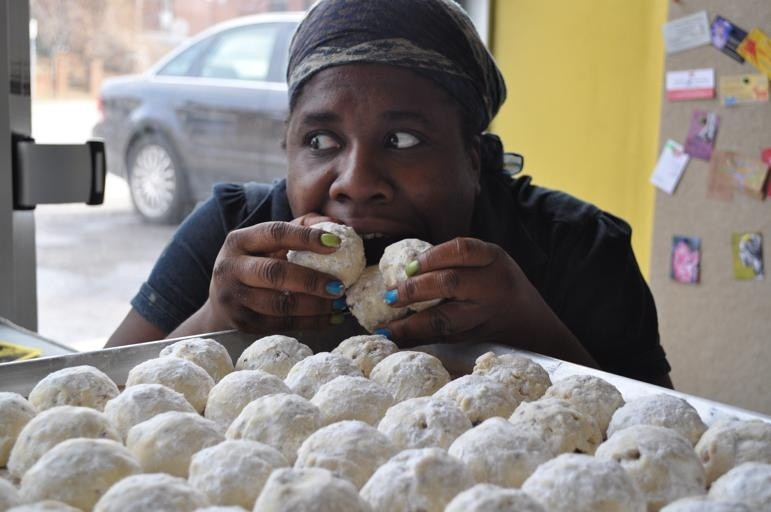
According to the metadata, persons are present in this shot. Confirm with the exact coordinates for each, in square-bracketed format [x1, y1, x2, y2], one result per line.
[102, 1, 674, 391]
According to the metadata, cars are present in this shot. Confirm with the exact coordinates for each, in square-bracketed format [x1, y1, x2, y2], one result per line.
[92, 12, 308, 226]
